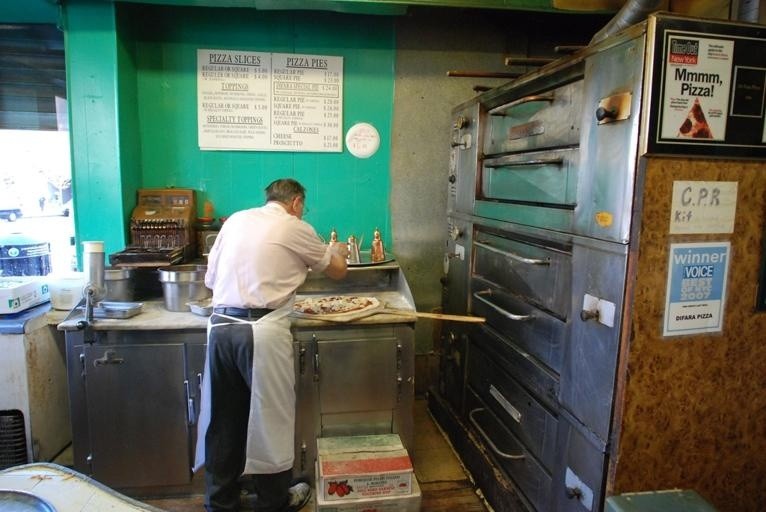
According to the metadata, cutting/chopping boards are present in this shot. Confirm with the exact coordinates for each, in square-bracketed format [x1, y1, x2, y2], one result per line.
[284, 294, 487, 323]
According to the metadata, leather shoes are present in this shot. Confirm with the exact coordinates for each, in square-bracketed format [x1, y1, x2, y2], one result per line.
[239, 482, 311, 512]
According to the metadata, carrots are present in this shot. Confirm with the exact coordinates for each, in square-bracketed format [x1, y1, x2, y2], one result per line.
[328, 482, 350, 497]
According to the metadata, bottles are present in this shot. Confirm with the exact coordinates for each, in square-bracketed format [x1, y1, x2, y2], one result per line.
[371, 228, 386, 263]
[346, 235, 361, 263]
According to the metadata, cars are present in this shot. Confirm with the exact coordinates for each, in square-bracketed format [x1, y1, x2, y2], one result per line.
[0, 208, 23, 222]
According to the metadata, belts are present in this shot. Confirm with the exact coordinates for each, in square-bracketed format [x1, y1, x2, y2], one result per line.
[214, 307, 276, 318]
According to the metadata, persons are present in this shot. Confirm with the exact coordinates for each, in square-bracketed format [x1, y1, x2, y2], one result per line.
[192, 179, 349, 512]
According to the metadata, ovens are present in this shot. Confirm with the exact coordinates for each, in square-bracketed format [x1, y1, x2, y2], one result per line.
[66, 330, 415, 499]
[426, 11, 647, 512]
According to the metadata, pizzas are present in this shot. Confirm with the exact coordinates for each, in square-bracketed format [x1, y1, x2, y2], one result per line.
[292, 295, 380, 316]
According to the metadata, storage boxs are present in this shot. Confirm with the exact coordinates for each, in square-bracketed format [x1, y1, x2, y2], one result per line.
[315, 437, 424, 511]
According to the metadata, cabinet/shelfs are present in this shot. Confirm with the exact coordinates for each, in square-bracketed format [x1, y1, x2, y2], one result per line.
[314, 330, 415, 460]
[65, 330, 193, 499]
